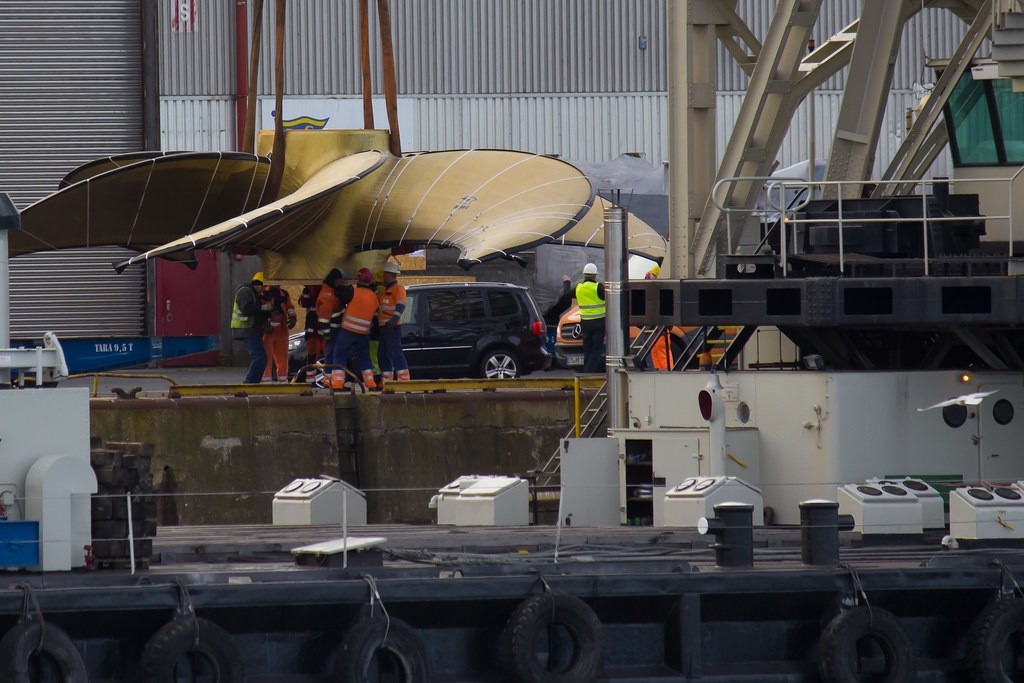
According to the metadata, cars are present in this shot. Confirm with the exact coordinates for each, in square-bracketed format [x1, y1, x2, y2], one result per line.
[556, 304, 741, 372]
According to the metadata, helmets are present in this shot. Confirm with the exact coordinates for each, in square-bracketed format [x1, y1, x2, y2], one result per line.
[330, 268, 342, 278]
[382, 262, 400, 273]
[357, 267, 372, 283]
[582, 262, 597, 274]
[251, 272, 264, 282]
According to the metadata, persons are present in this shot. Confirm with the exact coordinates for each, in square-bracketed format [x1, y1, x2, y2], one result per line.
[649, 267, 673, 369]
[315, 262, 377, 390]
[259, 284, 295, 383]
[298, 285, 326, 383]
[377, 262, 410, 381]
[229, 272, 273, 383]
[562, 263, 605, 372]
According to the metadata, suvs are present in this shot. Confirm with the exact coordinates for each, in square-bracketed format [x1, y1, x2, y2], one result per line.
[271, 279, 554, 386]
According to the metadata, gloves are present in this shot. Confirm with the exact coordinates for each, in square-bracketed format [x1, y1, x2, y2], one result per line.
[261, 303, 275, 311]
[286, 315, 296, 329]
[385, 320, 394, 329]
[302, 288, 311, 303]
[324, 333, 332, 339]
[261, 320, 272, 332]
